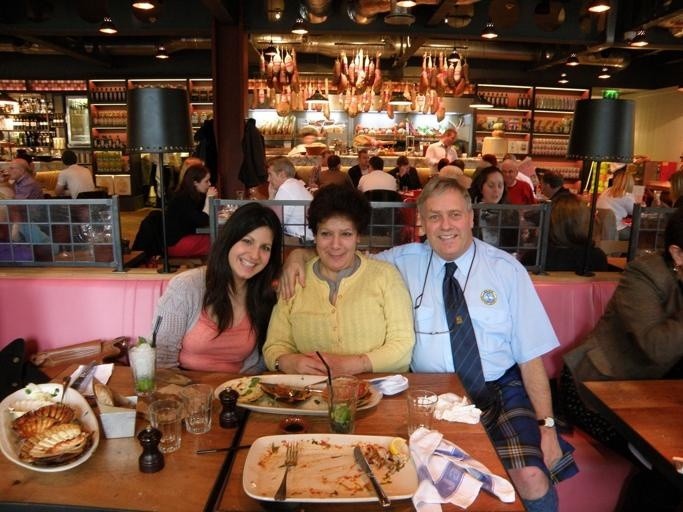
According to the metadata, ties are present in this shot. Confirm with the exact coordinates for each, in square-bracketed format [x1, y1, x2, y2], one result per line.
[439, 260, 493, 412]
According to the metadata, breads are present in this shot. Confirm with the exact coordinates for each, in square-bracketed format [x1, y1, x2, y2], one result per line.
[95, 383, 115, 406]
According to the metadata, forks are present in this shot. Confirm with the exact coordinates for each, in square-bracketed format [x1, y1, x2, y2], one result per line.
[271, 437, 301, 506]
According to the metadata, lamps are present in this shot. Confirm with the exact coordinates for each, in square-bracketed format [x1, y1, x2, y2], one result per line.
[264, 0, 278, 56]
[291, 22, 309, 35]
[566, 99, 636, 277]
[589, 0, 610, 13]
[481, 24, 498, 39]
[99, 18, 119, 34]
[448, 5, 460, 62]
[630, 30, 649, 46]
[396, 0, 416, 8]
[557, 72, 569, 84]
[566, 50, 580, 66]
[155, 45, 169, 59]
[597, 68, 611, 79]
[132, 0, 155, 10]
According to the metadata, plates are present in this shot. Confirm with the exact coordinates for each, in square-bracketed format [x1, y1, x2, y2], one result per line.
[1, 383, 100, 472]
[241, 432, 419, 503]
[214, 373, 383, 419]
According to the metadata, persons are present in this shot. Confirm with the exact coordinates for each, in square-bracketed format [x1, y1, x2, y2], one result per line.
[0, 148, 67, 261]
[260, 181, 416, 377]
[53, 150, 96, 199]
[156, 127, 682, 270]
[554, 204, 682, 511]
[274, 174, 568, 511]
[150, 201, 283, 374]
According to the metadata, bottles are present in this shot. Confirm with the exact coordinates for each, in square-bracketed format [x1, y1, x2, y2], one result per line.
[535, 97, 583, 112]
[478, 91, 533, 110]
[207, 186, 259, 222]
[192, 86, 214, 102]
[91, 85, 126, 174]
[475, 134, 529, 155]
[492, 117, 531, 132]
[219, 385, 242, 429]
[70, 101, 87, 114]
[537, 167, 580, 180]
[149, 186, 156, 206]
[1, 96, 65, 160]
[128, 341, 157, 397]
[328, 140, 376, 155]
[355, 121, 392, 135]
[532, 137, 569, 154]
[138, 424, 164, 474]
[126, 143, 196, 151]
[192, 112, 215, 128]
[533, 120, 561, 134]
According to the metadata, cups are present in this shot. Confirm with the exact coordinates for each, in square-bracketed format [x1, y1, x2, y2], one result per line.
[462, 153, 467, 158]
[150, 398, 183, 455]
[653, 190, 662, 206]
[178, 383, 214, 435]
[406, 387, 442, 443]
[279, 417, 309, 433]
[325, 375, 358, 435]
[403, 185, 409, 194]
[422, 144, 428, 157]
[99, 395, 138, 440]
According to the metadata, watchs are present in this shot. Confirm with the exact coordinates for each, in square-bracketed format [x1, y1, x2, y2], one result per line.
[536, 417, 554, 427]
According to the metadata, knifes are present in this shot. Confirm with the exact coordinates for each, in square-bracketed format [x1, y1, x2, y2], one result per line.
[352, 444, 391, 509]
[72, 357, 97, 390]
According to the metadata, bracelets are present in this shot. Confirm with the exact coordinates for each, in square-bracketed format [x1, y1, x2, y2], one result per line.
[273, 355, 283, 373]
[358, 353, 370, 372]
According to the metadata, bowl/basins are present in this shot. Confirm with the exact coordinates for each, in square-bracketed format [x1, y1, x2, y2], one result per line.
[305, 146, 326, 155]
[300, 152, 306, 155]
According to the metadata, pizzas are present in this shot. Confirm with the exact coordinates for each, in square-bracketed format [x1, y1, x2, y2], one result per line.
[230, 377, 264, 404]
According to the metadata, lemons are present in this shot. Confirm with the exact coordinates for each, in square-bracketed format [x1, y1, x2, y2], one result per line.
[390, 437, 406, 454]
